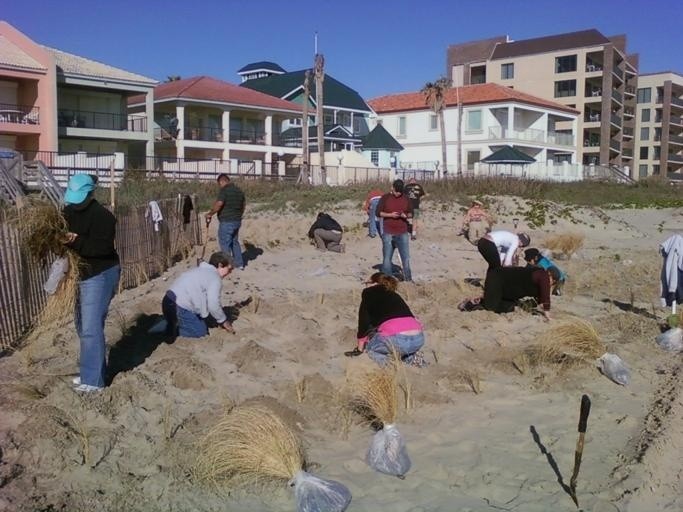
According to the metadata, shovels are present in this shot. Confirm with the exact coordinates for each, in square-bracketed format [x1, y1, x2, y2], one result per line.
[570, 394, 609, 509]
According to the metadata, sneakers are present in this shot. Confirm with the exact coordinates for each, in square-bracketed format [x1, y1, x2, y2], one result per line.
[72, 383, 105, 396]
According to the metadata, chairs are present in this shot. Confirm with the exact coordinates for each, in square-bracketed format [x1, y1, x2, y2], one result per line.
[22, 112, 38, 124]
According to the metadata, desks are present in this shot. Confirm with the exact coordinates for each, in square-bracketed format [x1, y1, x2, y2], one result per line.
[0, 110, 21, 122]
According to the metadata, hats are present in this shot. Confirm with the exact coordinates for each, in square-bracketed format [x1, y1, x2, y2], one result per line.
[392, 179, 404, 192]
[63, 173, 96, 204]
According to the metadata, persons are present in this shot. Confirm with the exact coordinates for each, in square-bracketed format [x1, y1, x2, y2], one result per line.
[147, 252, 234, 338]
[356, 272, 427, 368]
[363, 190, 385, 238]
[375, 179, 414, 283]
[204, 173, 245, 270]
[60, 172, 121, 393]
[308, 212, 346, 253]
[403, 178, 426, 240]
[458, 201, 568, 321]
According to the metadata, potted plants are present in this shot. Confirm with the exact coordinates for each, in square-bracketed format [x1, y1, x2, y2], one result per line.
[190, 112, 201, 140]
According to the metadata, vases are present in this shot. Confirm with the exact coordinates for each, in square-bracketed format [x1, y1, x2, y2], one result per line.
[215, 133, 222, 141]
